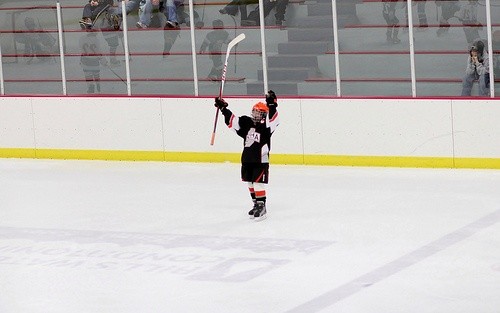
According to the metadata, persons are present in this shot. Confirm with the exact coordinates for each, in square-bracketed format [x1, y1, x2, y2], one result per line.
[219, 0, 259, 22]
[382, 0, 483, 45]
[241, 0, 289, 30]
[460, 40, 497, 96]
[214, 90, 280, 221]
[79, 0, 186, 30]
[11, 9, 239, 96]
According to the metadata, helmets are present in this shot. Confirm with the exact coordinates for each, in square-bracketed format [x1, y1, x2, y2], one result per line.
[251, 102, 270, 120]
[211, 19, 224, 30]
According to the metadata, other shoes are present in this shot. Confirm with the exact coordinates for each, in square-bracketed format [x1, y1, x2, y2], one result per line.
[136, 22, 147, 29]
[105, 13, 114, 26]
[274, 13, 286, 24]
[112, 15, 120, 31]
[219, 7, 238, 16]
[79, 18, 93, 27]
[80, 23, 89, 31]
[166, 21, 177, 28]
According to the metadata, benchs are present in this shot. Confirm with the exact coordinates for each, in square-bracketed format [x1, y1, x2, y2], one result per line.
[297, 0, 500, 97]
[0, 0, 269, 98]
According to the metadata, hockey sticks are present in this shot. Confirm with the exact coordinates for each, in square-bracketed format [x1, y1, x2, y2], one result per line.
[230, 15, 237, 74]
[209, 31, 247, 146]
[199, 0, 206, 29]
[108, 67, 139, 87]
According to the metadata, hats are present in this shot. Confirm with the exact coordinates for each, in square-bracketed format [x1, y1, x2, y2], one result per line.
[472, 39, 485, 52]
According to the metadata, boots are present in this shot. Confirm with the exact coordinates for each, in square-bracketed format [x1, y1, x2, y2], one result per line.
[207, 64, 226, 83]
[393, 25, 402, 45]
[253, 197, 267, 221]
[87, 82, 100, 94]
[437, 16, 449, 37]
[109, 47, 120, 66]
[405, 13, 409, 28]
[385, 26, 394, 43]
[417, 18, 429, 28]
[248, 193, 257, 218]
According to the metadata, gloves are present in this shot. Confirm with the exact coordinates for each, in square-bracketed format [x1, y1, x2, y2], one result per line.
[266, 90, 278, 109]
[214, 96, 228, 112]
